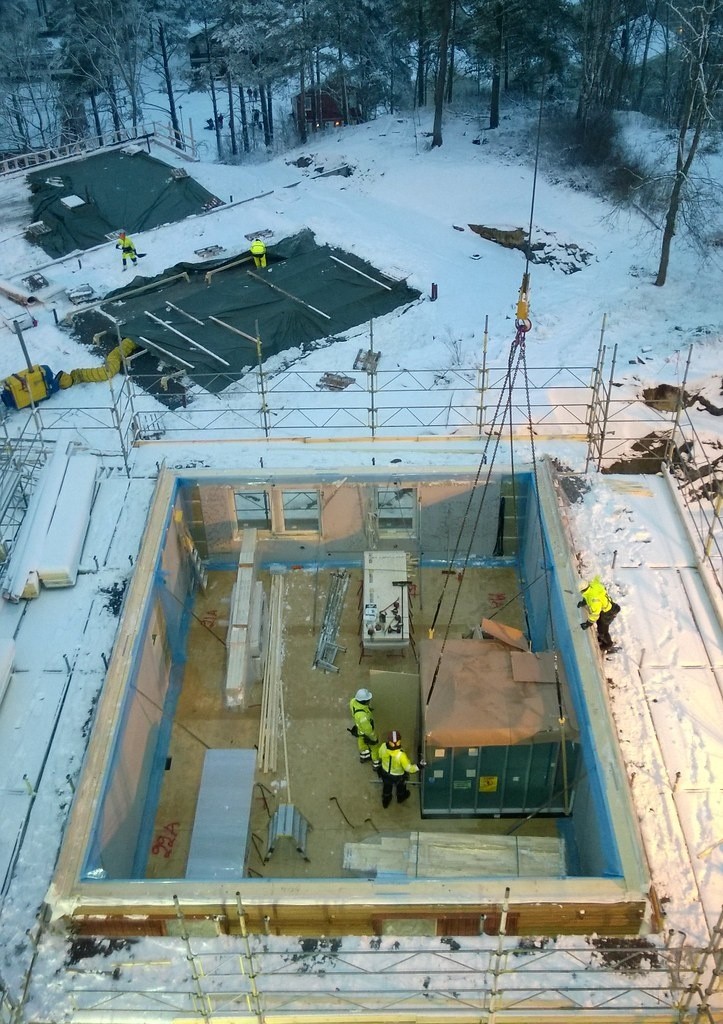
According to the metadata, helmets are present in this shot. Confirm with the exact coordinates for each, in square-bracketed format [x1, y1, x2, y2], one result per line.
[576, 580, 588, 591]
[356, 689, 372, 701]
[386, 731, 401, 746]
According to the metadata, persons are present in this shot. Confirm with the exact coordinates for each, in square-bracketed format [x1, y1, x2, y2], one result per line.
[218, 113, 223, 129]
[246, 87, 263, 130]
[206, 117, 214, 131]
[115, 232, 138, 271]
[378, 729, 428, 810]
[348, 688, 382, 773]
[576, 579, 622, 653]
[250, 238, 267, 269]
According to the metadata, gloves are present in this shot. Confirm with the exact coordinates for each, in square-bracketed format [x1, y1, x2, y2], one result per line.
[581, 620, 593, 630]
[133, 249, 137, 255]
[577, 599, 586, 608]
[116, 245, 119, 248]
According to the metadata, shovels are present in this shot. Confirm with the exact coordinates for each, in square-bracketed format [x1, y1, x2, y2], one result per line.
[117, 247, 147, 258]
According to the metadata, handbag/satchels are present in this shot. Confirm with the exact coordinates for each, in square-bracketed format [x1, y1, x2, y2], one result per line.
[347, 725, 357, 736]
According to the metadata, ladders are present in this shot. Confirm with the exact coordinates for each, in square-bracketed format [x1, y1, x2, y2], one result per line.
[174, 510, 209, 596]
[264, 803, 313, 862]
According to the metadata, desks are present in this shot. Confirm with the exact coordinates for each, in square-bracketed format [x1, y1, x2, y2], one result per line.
[185, 748, 274, 879]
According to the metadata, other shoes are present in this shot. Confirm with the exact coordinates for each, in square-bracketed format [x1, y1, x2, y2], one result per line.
[382, 797, 392, 808]
[597, 636, 601, 642]
[397, 791, 410, 803]
[600, 643, 613, 649]
